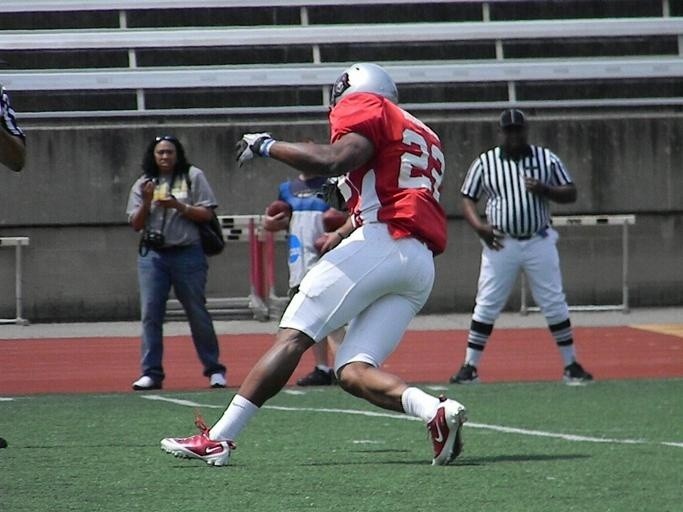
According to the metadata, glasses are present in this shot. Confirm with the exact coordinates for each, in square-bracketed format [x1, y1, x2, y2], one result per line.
[155, 135, 179, 141]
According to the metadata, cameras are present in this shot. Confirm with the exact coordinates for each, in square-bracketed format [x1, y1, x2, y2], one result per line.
[143, 231, 165, 249]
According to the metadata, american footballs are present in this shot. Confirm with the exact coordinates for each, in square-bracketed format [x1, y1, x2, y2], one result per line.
[315, 235, 330, 253]
[268, 200, 292, 222]
[321, 211, 344, 231]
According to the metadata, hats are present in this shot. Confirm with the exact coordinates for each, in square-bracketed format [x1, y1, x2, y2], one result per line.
[496, 108, 528, 133]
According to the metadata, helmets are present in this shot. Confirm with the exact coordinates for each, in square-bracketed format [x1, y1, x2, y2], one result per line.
[329, 63, 398, 107]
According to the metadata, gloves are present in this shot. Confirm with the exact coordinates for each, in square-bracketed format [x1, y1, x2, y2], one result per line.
[234, 131, 276, 168]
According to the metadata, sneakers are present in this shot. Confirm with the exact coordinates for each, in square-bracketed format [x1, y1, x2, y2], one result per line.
[425, 395, 468, 466]
[563, 362, 592, 383]
[132, 375, 162, 389]
[160, 430, 236, 466]
[449, 362, 480, 384]
[296, 366, 332, 385]
[210, 373, 227, 387]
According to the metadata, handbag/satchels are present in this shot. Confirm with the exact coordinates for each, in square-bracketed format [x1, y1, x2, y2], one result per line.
[200, 212, 225, 257]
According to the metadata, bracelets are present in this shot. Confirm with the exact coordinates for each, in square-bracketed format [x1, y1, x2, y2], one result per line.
[181, 206, 194, 215]
[336, 230, 344, 240]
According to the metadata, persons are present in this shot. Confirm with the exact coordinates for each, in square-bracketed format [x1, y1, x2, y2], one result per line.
[2, 84, 27, 448]
[160, 64, 468, 468]
[126, 137, 227, 390]
[453, 109, 595, 389]
[262, 169, 362, 386]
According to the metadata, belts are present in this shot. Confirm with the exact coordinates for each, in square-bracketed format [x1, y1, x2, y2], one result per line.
[512, 220, 553, 240]
